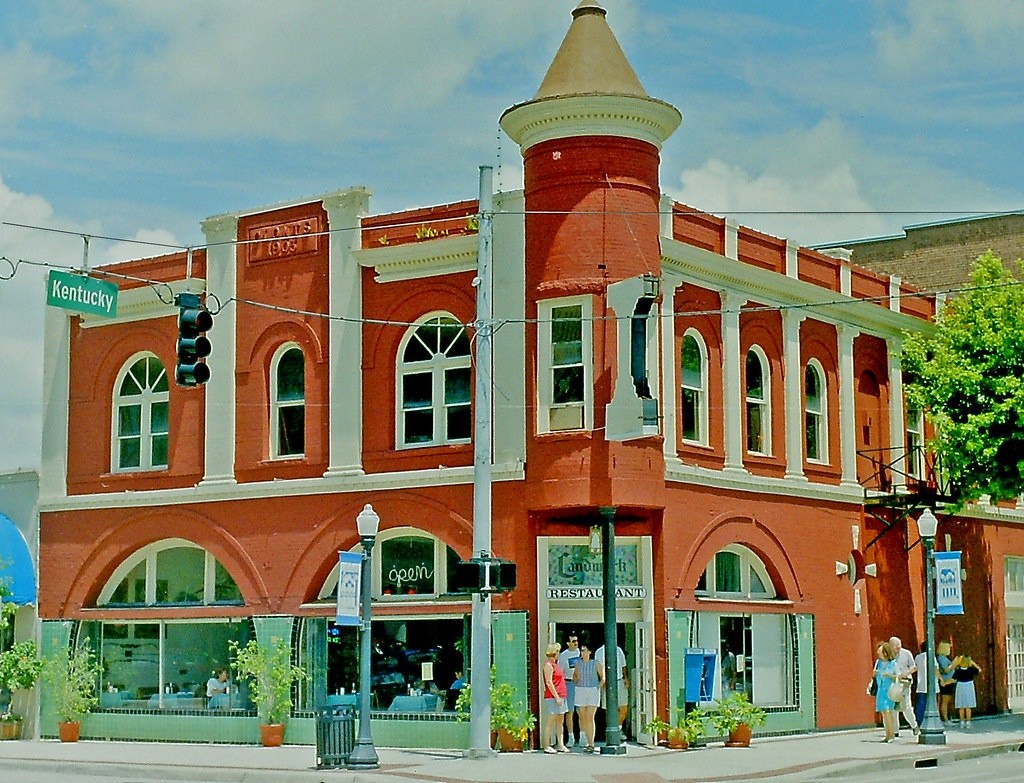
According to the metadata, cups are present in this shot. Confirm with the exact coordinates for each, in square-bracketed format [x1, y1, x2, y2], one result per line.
[226, 687, 229, 694]
[165, 687, 169, 694]
[109, 686, 113, 693]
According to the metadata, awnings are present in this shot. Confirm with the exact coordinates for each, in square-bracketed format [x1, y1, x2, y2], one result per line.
[0, 512, 37, 608]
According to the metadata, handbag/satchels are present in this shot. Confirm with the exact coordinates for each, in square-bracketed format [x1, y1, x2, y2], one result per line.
[871, 675, 878, 696]
[886, 675, 903, 703]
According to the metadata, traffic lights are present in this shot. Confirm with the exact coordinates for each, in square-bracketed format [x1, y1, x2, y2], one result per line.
[176, 293, 211, 387]
[456, 562, 487, 590]
[490, 562, 516, 590]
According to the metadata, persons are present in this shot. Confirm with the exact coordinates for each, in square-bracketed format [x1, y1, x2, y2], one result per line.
[543, 635, 630, 753]
[414, 667, 468, 696]
[873, 637, 982, 743]
[206, 668, 230, 701]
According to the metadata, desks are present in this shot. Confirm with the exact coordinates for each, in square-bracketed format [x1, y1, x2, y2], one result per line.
[211, 692, 242, 709]
[151, 692, 193, 708]
[325, 693, 374, 710]
[390, 695, 444, 712]
[100, 690, 133, 706]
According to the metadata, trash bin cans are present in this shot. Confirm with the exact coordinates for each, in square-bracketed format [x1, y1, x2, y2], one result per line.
[316, 704, 355, 770]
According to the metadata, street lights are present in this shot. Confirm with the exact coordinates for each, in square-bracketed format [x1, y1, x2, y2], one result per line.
[917, 507, 951, 747]
[346, 503, 381, 770]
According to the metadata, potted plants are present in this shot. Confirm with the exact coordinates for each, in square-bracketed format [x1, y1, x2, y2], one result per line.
[42, 633, 106, 742]
[712, 692, 768, 746]
[0, 638, 47, 740]
[640, 698, 706, 750]
[453, 666, 537, 751]
[225, 635, 309, 747]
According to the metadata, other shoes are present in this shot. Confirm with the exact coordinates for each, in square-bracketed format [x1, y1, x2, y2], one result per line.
[945, 721, 954, 727]
[882, 734, 896, 743]
[584, 746, 595, 753]
[960, 724, 964, 730]
[618, 729, 626, 741]
[913, 727, 919, 735]
[966, 723, 971, 729]
[543, 746, 556, 754]
[556, 746, 570, 752]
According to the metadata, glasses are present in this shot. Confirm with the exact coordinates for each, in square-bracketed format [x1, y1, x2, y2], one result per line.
[569, 640, 578, 644]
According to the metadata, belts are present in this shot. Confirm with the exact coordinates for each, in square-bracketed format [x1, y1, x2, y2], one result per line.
[565, 678, 575, 683]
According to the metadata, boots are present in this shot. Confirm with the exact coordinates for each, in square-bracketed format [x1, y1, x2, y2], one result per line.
[565, 733, 575, 748]
[579, 731, 588, 747]
